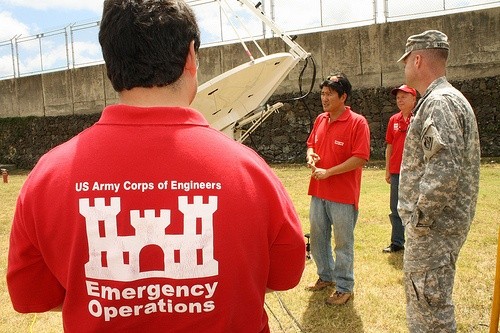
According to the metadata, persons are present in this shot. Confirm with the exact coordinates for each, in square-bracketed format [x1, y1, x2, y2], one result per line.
[305, 74, 371, 305]
[5, 0, 307, 333]
[395, 29, 479, 333]
[381, 82, 419, 254]
